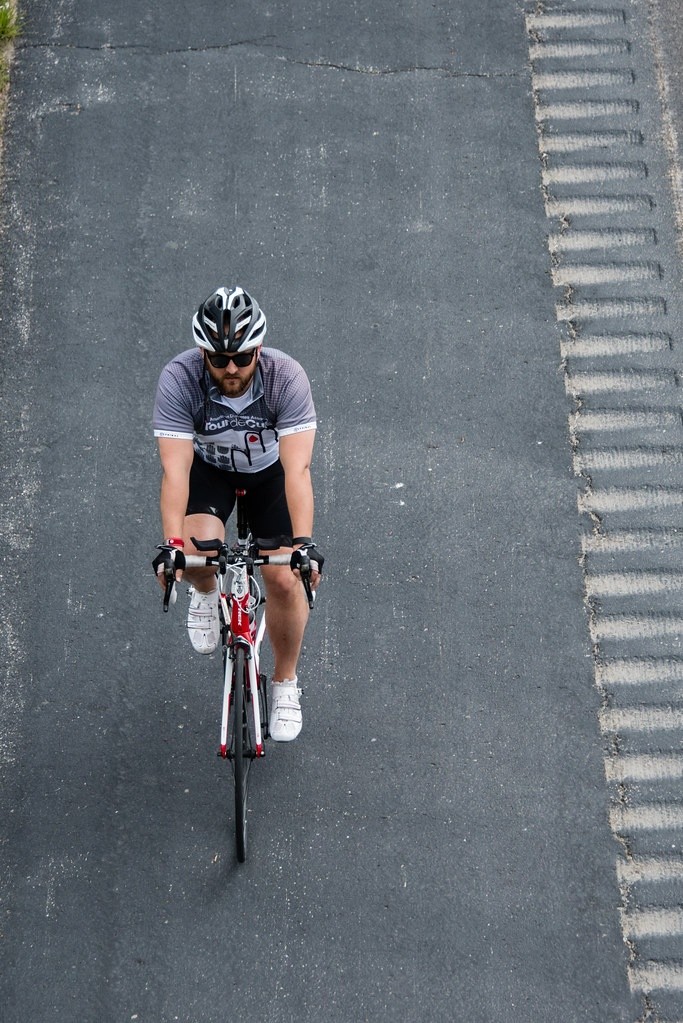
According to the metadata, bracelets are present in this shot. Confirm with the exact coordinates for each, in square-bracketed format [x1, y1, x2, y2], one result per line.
[165, 538, 185, 548]
[293, 537, 313, 545]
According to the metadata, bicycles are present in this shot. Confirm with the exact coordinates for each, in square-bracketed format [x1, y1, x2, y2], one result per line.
[155, 482, 321, 862]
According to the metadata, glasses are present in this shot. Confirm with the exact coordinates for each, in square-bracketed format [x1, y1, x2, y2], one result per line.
[206, 347, 256, 368]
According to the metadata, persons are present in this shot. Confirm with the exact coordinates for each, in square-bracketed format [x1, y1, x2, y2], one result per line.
[152, 288, 325, 742]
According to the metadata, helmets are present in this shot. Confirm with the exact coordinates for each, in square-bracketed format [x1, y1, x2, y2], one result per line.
[192, 286, 267, 356]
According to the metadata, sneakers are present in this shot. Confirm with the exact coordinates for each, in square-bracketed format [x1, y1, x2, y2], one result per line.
[187, 575, 220, 654]
[269, 675, 302, 741]
